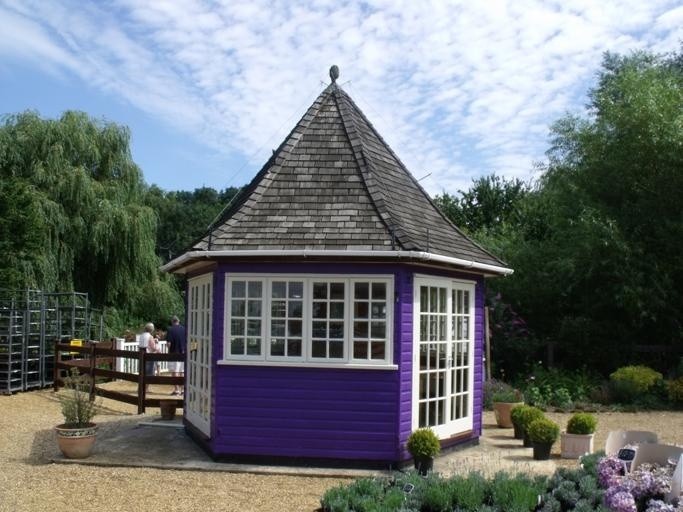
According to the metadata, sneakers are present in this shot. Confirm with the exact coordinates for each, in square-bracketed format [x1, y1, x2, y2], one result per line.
[170, 390, 184, 394]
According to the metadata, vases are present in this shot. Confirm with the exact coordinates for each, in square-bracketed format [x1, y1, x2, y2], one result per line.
[160, 401, 178, 420]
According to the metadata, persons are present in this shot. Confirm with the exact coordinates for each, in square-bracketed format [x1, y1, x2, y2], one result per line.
[165, 315, 186, 396]
[155, 338, 161, 377]
[136, 322, 157, 394]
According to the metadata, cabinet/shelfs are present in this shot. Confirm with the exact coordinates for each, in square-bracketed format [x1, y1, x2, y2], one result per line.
[0, 285, 103, 395]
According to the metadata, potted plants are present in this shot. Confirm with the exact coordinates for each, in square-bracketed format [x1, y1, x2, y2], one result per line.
[406, 390, 596, 475]
[53, 367, 105, 458]
